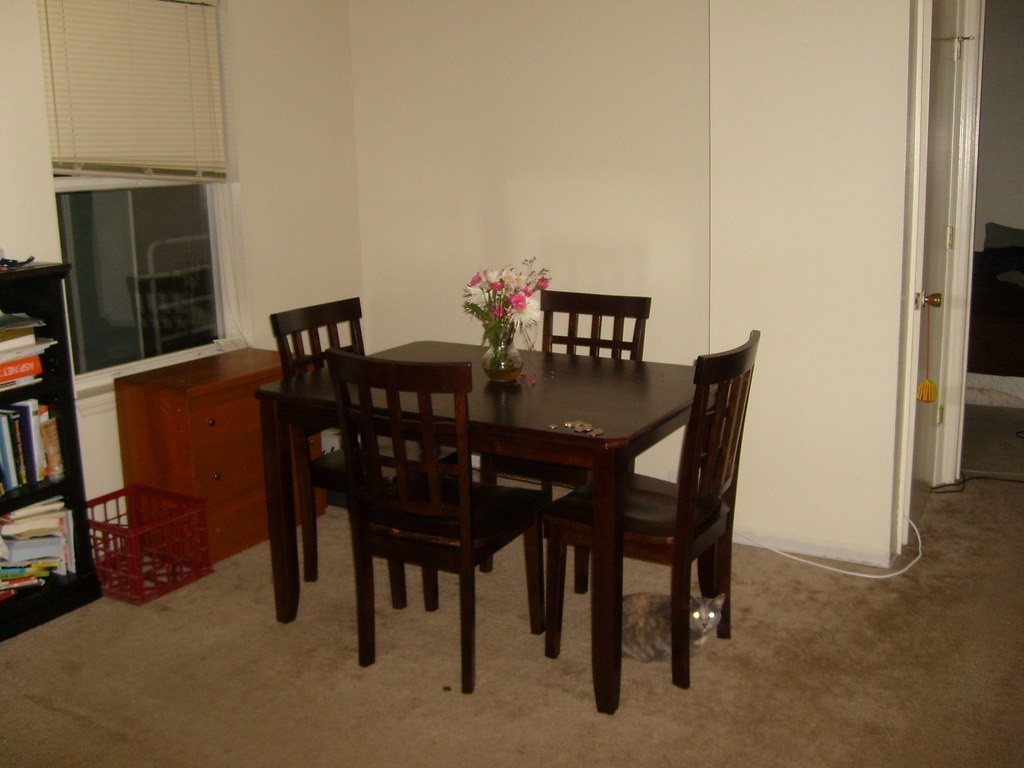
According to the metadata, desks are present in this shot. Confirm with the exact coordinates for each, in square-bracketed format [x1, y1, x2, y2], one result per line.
[253, 340, 733, 714]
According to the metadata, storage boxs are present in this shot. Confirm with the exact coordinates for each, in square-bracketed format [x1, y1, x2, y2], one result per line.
[86, 485, 213, 605]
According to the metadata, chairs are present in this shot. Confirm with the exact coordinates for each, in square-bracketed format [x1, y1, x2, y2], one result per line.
[478, 290, 652, 595]
[270, 297, 458, 608]
[541, 331, 759, 690]
[324, 350, 545, 695]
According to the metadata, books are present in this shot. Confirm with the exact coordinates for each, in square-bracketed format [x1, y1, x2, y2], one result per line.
[0, 311, 58, 394]
[0, 497, 78, 602]
[0, 397, 66, 495]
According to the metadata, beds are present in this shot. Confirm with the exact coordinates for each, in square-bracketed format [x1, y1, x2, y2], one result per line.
[967, 222, 1024, 377]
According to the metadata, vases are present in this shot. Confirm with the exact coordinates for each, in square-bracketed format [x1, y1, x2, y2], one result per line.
[482, 340, 523, 384]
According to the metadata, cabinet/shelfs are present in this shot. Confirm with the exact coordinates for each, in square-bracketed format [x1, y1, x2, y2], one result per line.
[115, 346, 328, 572]
[0, 261, 103, 642]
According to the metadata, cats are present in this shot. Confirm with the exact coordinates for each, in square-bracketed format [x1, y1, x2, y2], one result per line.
[621, 592, 726, 664]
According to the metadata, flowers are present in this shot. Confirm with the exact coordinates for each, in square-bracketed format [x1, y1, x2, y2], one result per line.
[462, 256, 552, 340]
